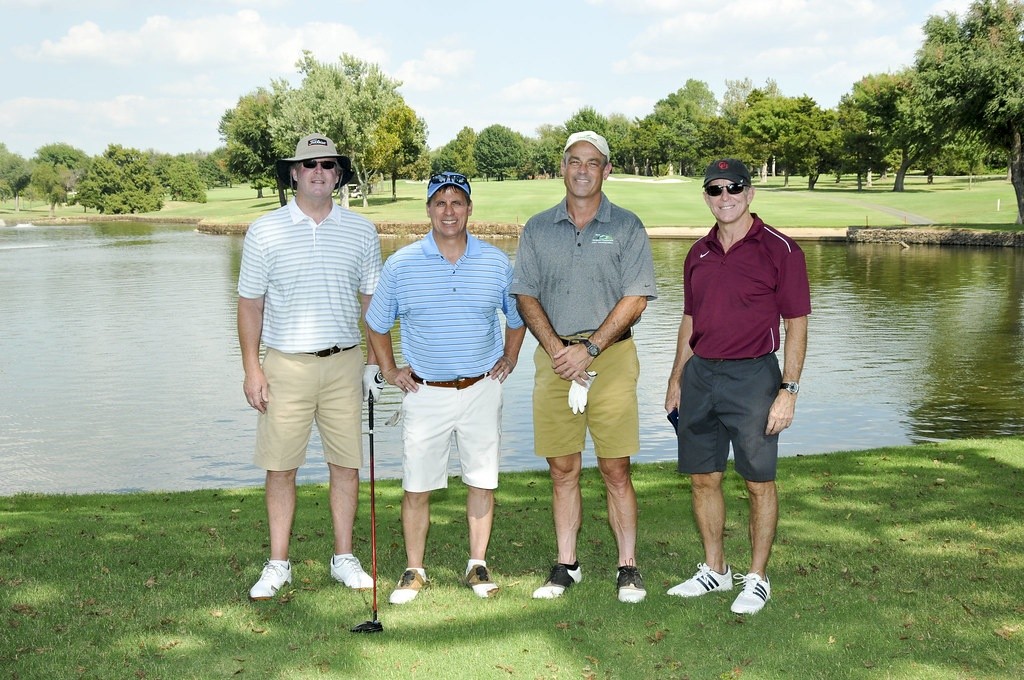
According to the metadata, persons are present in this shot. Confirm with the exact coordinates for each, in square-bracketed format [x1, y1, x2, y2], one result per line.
[664, 158, 811, 615]
[237, 133, 385, 600]
[364, 171, 528, 604]
[509, 130, 658, 603]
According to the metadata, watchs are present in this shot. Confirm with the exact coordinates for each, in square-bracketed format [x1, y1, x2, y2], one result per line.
[583, 340, 601, 357]
[780, 382, 799, 394]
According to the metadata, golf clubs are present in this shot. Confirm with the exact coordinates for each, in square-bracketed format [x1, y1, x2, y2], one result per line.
[348, 390, 383, 635]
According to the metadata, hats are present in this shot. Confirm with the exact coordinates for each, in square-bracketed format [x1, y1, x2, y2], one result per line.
[702, 159, 751, 187]
[276, 133, 355, 189]
[426, 172, 470, 198]
[564, 130, 609, 163]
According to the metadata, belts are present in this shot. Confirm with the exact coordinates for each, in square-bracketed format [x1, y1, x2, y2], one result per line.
[410, 372, 491, 390]
[560, 327, 631, 348]
[305, 346, 357, 358]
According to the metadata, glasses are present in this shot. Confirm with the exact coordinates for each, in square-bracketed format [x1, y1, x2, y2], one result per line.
[705, 183, 750, 196]
[297, 159, 339, 169]
[428, 174, 471, 194]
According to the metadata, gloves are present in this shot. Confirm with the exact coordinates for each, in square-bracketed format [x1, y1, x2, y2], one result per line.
[363, 363, 386, 403]
[567, 371, 597, 414]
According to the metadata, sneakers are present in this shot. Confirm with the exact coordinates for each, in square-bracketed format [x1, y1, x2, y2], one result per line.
[390, 568, 425, 604]
[330, 556, 374, 591]
[731, 570, 772, 615]
[249, 556, 292, 601]
[667, 562, 733, 597]
[465, 564, 499, 599]
[532, 562, 582, 601]
[616, 558, 646, 604]
